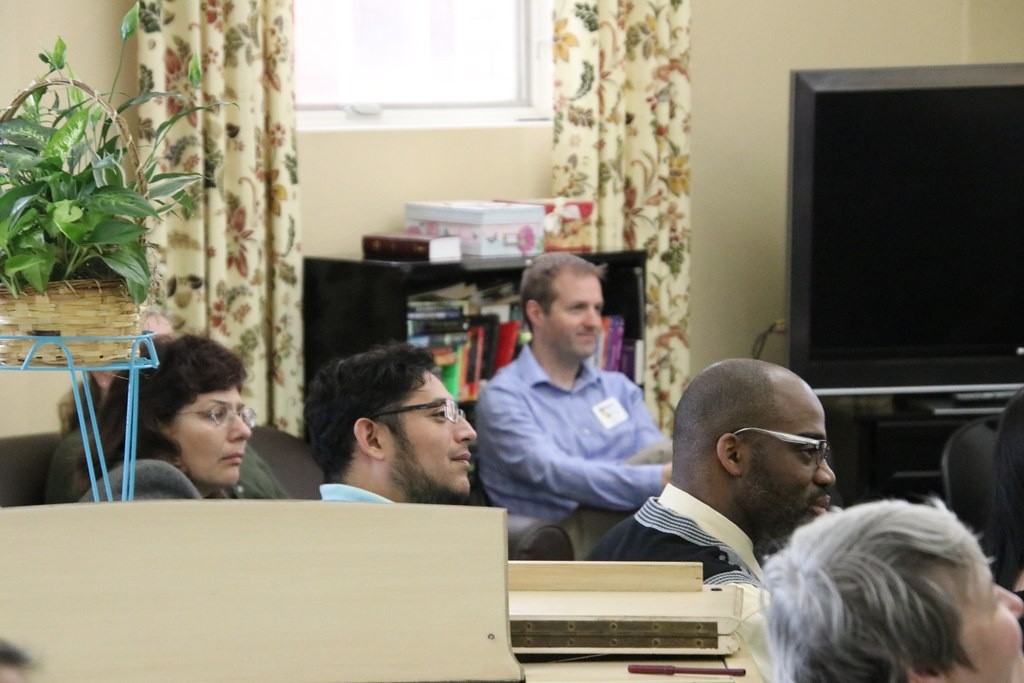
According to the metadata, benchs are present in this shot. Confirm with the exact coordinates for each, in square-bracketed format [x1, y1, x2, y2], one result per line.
[0, 426, 327, 508]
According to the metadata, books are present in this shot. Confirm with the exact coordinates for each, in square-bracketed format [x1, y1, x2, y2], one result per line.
[362, 233, 461, 260]
[584, 315, 644, 385]
[406, 284, 532, 401]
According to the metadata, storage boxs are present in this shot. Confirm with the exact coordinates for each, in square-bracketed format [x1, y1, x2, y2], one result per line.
[406, 196, 594, 258]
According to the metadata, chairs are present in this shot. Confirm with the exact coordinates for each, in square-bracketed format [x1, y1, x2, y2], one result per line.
[506, 514, 575, 562]
[942, 415, 1003, 503]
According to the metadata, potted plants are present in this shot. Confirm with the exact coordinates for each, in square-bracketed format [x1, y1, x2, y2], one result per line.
[0, 2, 241, 369]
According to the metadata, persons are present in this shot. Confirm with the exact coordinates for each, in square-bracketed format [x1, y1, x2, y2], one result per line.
[476, 249, 672, 559]
[45, 310, 290, 502]
[975, 385, 1024, 626]
[761, 498, 1024, 683]
[77, 335, 252, 502]
[583, 358, 836, 683]
[301, 344, 476, 503]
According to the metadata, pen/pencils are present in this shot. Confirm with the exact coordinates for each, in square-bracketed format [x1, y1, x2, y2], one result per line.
[626, 663, 746, 676]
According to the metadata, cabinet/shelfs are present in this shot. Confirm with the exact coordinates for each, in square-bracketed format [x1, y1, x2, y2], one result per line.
[303, 257, 645, 442]
[825, 397, 1004, 505]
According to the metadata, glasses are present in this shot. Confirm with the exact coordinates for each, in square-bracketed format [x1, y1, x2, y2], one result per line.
[733, 427, 830, 467]
[161, 405, 257, 428]
[370, 398, 466, 425]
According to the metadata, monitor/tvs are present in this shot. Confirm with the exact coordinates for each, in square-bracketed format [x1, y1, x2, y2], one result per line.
[787, 62, 1024, 414]
